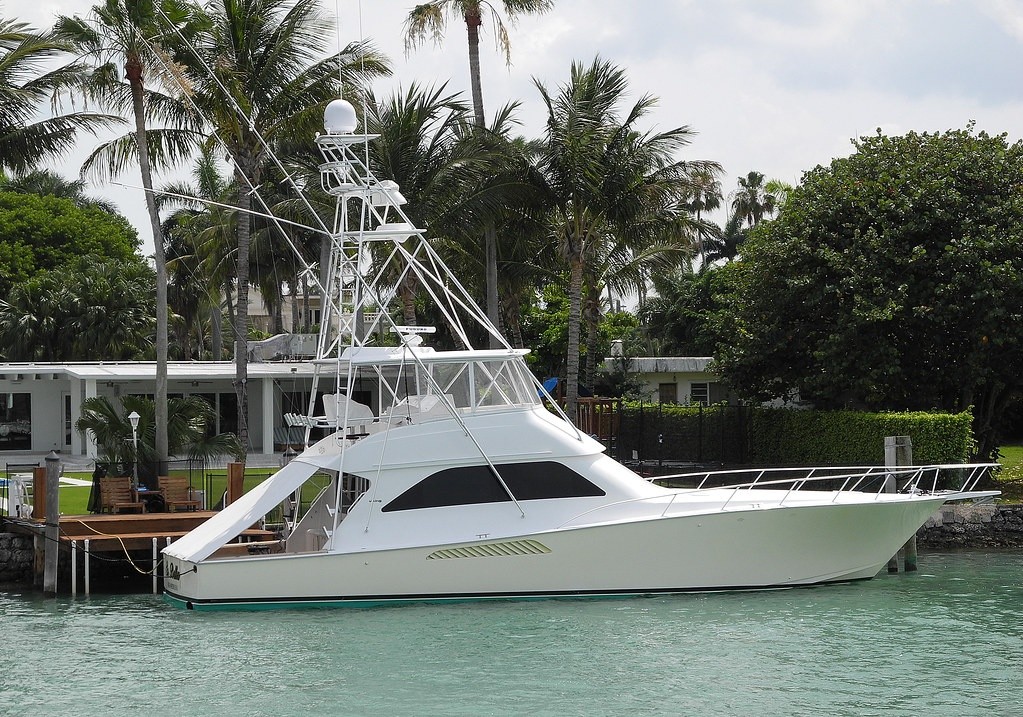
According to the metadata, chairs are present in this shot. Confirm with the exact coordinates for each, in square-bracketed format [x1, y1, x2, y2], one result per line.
[273, 427, 319, 452]
[157, 474, 202, 513]
[99, 477, 146, 515]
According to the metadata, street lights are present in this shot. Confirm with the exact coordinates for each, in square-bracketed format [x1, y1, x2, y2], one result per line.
[123, 409, 141, 503]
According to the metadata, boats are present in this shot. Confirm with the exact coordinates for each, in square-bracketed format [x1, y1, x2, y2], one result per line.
[110, 0, 1005, 609]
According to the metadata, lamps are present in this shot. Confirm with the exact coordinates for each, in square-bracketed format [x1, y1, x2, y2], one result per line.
[291, 367, 298, 373]
[107, 381, 113, 388]
[191, 379, 199, 387]
[274, 379, 281, 386]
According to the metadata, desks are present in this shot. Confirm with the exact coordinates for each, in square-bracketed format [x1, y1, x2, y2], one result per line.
[133, 491, 160, 514]
